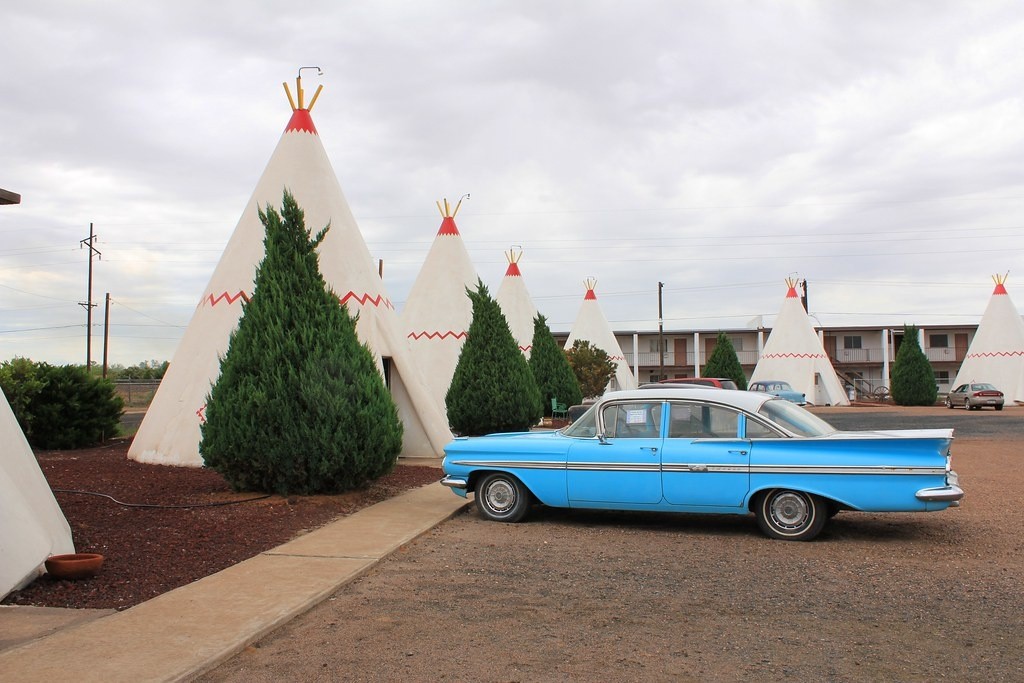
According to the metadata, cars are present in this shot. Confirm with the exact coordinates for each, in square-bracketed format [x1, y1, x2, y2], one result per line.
[944, 382, 1006, 411]
[567, 376, 739, 425]
[438, 386, 965, 542]
[748, 380, 807, 406]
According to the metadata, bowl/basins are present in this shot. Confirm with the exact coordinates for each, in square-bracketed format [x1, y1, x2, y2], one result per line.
[45, 554, 103, 578]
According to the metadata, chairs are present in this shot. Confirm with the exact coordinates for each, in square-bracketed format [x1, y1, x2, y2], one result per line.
[550, 396, 568, 422]
[649, 404, 675, 431]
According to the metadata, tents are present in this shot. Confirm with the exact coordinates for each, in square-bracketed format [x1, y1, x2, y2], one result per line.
[747, 277, 852, 407]
[0, 385, 75, 604]
[399, 193, 638, 437]
[949, 270, 1024, 407]
[126, 67, 455, 466]
